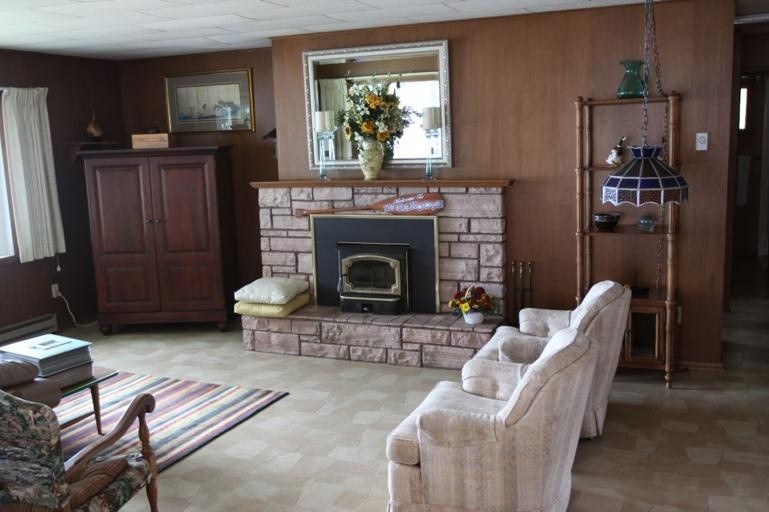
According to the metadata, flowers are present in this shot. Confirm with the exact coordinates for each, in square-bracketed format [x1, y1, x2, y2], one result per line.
[447, 284, 499, 314]
[333, 71, 421, 149]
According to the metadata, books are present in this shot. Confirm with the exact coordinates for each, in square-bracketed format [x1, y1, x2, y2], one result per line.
[0, 333, 96, 376]
[0, 361, 94, 390]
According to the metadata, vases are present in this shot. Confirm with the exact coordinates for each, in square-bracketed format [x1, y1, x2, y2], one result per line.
[464, 313, 485, 326]
[356, 139, 385, 180]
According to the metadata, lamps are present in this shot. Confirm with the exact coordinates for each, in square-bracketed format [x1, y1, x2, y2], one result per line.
[600, 1, 692, 206]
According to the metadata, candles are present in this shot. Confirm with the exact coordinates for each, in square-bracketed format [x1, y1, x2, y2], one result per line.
[314, 111, 326, 132]
[434, 106, 442, 128]
[325, 110, 334, 133]
[422, 106, 436, 129]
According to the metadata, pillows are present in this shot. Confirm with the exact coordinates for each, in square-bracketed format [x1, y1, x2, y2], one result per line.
[498, 333, 550, 364]
[233, 294, 313, 318]
[234, 275, 309, 303]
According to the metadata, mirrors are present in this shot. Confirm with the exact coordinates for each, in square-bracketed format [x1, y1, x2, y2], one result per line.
[300, 38, 455, 170]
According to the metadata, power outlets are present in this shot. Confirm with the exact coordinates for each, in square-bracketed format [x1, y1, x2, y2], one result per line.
[51, 282, 59, 298]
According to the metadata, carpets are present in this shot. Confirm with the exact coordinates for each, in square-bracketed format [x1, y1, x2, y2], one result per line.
[56, 366, 290, 477]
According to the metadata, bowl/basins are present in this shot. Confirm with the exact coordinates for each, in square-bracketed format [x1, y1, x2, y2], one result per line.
[592, 212, 621, 231]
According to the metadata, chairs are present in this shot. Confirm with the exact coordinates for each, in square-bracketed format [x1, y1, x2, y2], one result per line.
[385, 328, 597, 512]
[463, 282, 633, 441]
[1, 387, 160, 512]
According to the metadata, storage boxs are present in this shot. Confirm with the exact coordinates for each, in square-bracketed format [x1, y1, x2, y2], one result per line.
[131, 133, 175, 149]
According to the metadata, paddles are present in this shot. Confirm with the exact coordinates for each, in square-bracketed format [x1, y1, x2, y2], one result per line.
[295, 193, 446, 217]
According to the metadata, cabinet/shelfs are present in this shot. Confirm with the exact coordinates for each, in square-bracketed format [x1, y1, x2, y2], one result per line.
[572, 88, 684, 391]
[75, 145, 230, 334]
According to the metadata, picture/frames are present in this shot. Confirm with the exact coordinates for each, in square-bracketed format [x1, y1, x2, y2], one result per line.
[162, 68, 256, 135]
